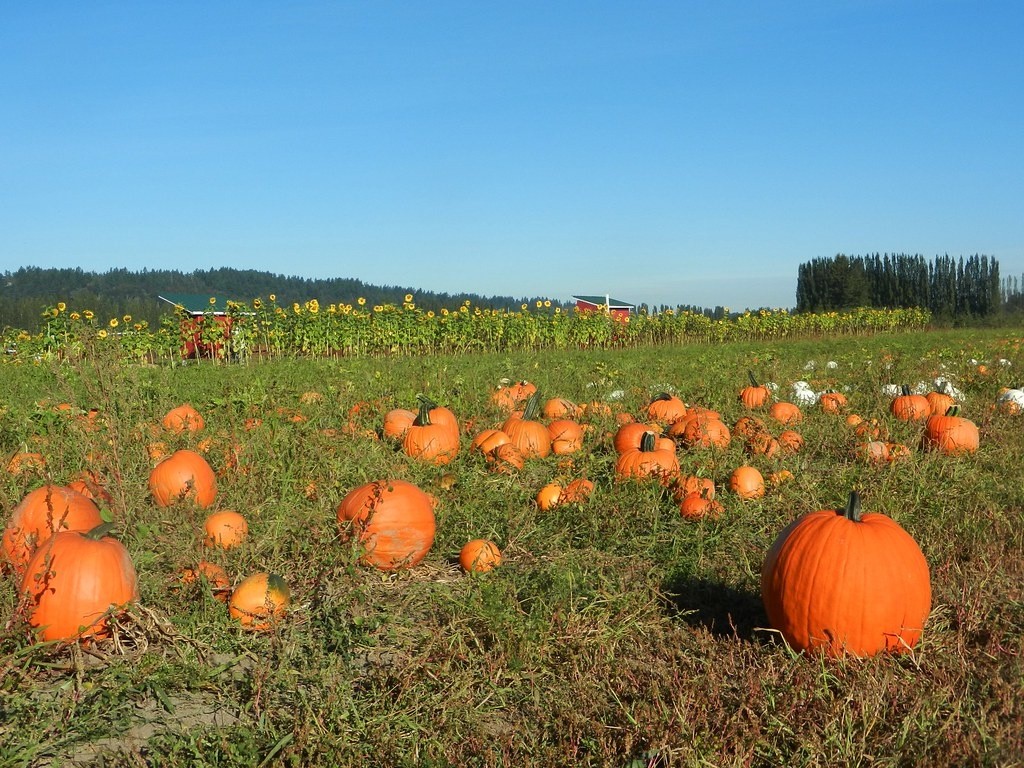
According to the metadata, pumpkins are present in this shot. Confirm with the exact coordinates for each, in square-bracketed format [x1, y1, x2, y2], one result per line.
[1, 365, 1017, 657]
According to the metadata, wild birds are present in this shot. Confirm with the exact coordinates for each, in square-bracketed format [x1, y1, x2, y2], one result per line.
[588, 354, 1024, 421]
